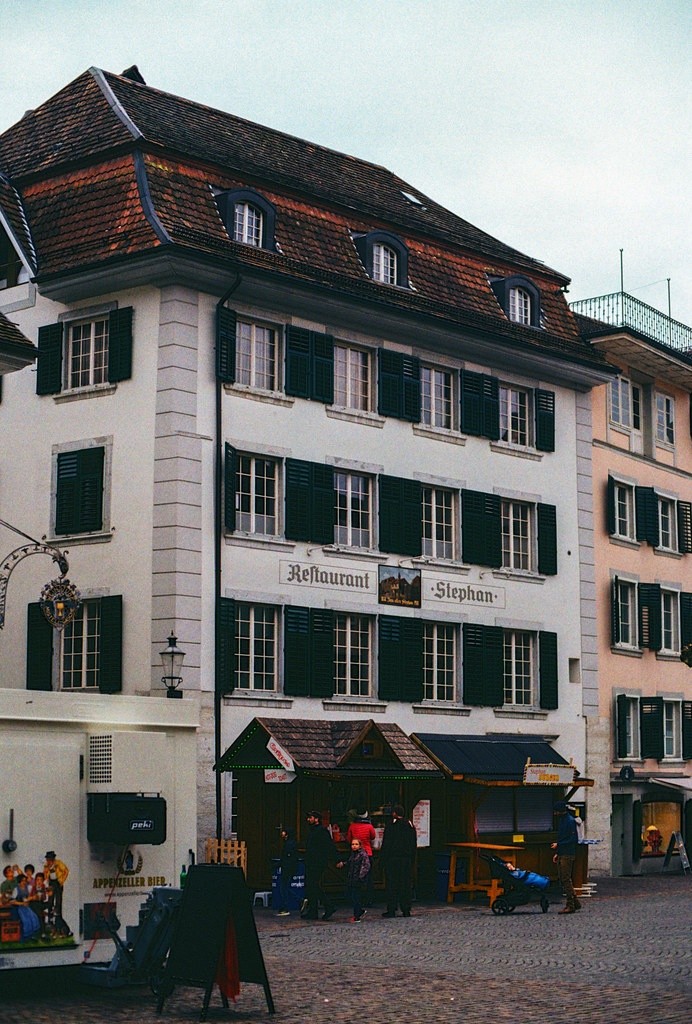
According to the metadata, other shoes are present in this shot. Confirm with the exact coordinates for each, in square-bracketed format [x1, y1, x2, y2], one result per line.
[271, 898, 413, 923]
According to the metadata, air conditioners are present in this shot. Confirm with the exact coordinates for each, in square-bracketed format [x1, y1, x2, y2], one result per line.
[85, 729, 167, 795]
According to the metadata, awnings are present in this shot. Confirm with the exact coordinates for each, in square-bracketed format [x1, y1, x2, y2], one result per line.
[650, 776, 691, 793]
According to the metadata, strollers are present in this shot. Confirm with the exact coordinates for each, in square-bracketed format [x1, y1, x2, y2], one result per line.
[478, 852, 551, 916]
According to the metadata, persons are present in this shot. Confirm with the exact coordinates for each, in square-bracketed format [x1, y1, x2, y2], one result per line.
[301, 809, 344, 920]
[377, 805, 417, 919]
[275, 824, 308, 916]
[346, 806, 375, 906]
[550, 801, 582, 914]
[335, 838, 371, 923]
[504, 862, 549, 890]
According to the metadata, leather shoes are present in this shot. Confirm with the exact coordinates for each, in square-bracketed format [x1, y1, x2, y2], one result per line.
[557, 906, 576, 915]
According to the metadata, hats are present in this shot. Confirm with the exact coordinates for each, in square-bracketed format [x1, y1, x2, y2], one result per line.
[554, 801, 566, 811]
[357, 808, 368, 819]
[303, 810, 322, 819]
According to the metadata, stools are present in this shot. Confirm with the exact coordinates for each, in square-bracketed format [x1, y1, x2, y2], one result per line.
[253, 891, 272, 907]
